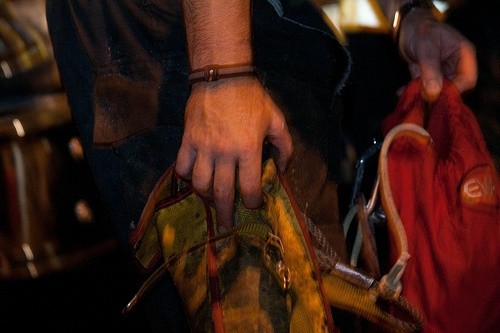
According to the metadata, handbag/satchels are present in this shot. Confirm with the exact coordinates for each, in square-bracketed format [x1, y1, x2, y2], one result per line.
[122, 127, 424, 333]
[368, 75, 500, 333]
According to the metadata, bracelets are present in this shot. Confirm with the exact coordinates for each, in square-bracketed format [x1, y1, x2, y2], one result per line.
[390, 1, 432, 44]
[187, 62, 255, 84]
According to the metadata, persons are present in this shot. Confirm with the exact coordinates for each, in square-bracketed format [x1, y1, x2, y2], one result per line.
[45, 1, 477, 333]
